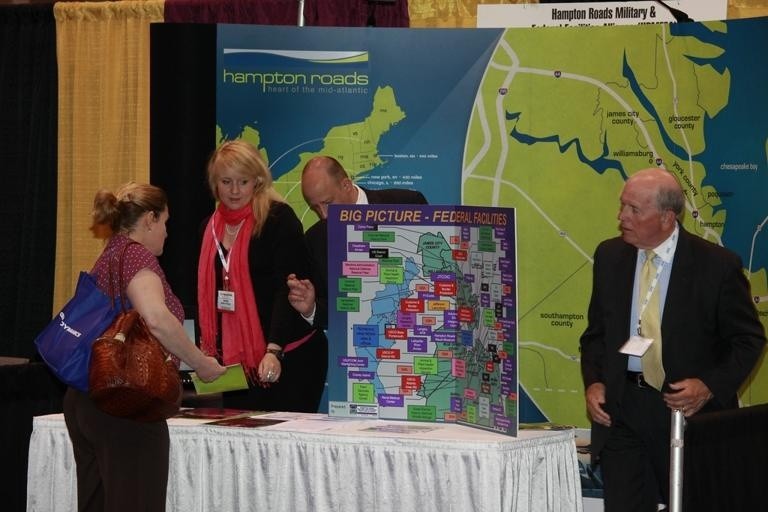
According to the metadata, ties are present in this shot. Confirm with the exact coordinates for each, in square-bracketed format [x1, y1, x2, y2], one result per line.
[638, 249, 666, 392]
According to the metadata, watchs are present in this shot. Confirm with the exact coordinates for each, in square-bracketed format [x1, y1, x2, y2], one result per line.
[267, 348, 285, 360]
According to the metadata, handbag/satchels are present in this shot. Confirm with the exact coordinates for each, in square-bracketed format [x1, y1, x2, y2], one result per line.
[34, 271, 183, 422]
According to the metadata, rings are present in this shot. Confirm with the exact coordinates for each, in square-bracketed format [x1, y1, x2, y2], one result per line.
[267, 369, 275, 381]
[682, 405, 685, 412]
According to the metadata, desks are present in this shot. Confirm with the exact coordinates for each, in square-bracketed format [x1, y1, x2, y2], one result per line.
[27, 407, 583, 511]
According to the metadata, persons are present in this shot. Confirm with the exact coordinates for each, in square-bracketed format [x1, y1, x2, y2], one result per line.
[286, 155, 429, 411]
[193, 141, 328, 413]
[579, 168, 765, 512]
[62, 181, 227, 512]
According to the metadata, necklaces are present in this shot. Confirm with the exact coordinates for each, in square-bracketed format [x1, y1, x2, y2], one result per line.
[226, 222, 238, 235]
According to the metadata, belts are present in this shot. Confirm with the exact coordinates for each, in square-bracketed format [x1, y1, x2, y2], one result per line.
[627, 371, 656, 390]
